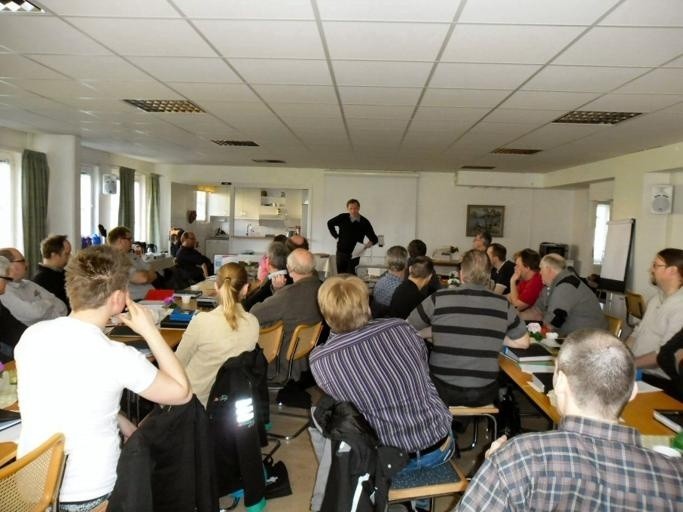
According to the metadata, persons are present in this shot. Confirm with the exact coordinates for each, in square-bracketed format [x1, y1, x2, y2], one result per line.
[1, 226, 323, 409]
[326, 198, 378, 276]
[453, 328, 683, 512]
[305, 274, 454, 512]
[374, 230, 607, 441]
[627, 248, 683, 384]
[12, 246, 193, 512]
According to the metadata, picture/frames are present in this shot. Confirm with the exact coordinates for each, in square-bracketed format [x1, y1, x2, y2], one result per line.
[466, 204, 505, 238]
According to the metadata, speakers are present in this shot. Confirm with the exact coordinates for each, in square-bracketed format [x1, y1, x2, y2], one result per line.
[102, 174, 116, 195]
[649, 184, 674, 215]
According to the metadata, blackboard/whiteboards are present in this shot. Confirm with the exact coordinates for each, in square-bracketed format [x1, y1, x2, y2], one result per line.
[600, 220, 633, 282]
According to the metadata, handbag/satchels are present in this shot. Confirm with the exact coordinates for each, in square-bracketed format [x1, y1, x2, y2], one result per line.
[263, 459, 293, 500]
[491, 385, 521, 434]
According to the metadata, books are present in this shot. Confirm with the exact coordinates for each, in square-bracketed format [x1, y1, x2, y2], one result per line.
[0, 410, 21, 430]
[652, 409, 683, 435]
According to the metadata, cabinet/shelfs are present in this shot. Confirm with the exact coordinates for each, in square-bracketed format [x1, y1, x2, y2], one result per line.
[233, 188, 262, 221]
[261, 196, 287, 221]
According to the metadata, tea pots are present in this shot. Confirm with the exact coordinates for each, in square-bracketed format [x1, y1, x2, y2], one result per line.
[146, 244, 157, 253]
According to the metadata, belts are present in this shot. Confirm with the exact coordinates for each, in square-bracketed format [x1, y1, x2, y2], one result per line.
[408, 433, 448, 460]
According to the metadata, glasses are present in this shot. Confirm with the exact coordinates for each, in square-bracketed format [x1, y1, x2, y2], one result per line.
[653, 262, 666, 269]
[10, 259, 25, 262]
[188, 237, 195, 240]
[121, 237, 131, 241]
[0, 277, 14, 281]
